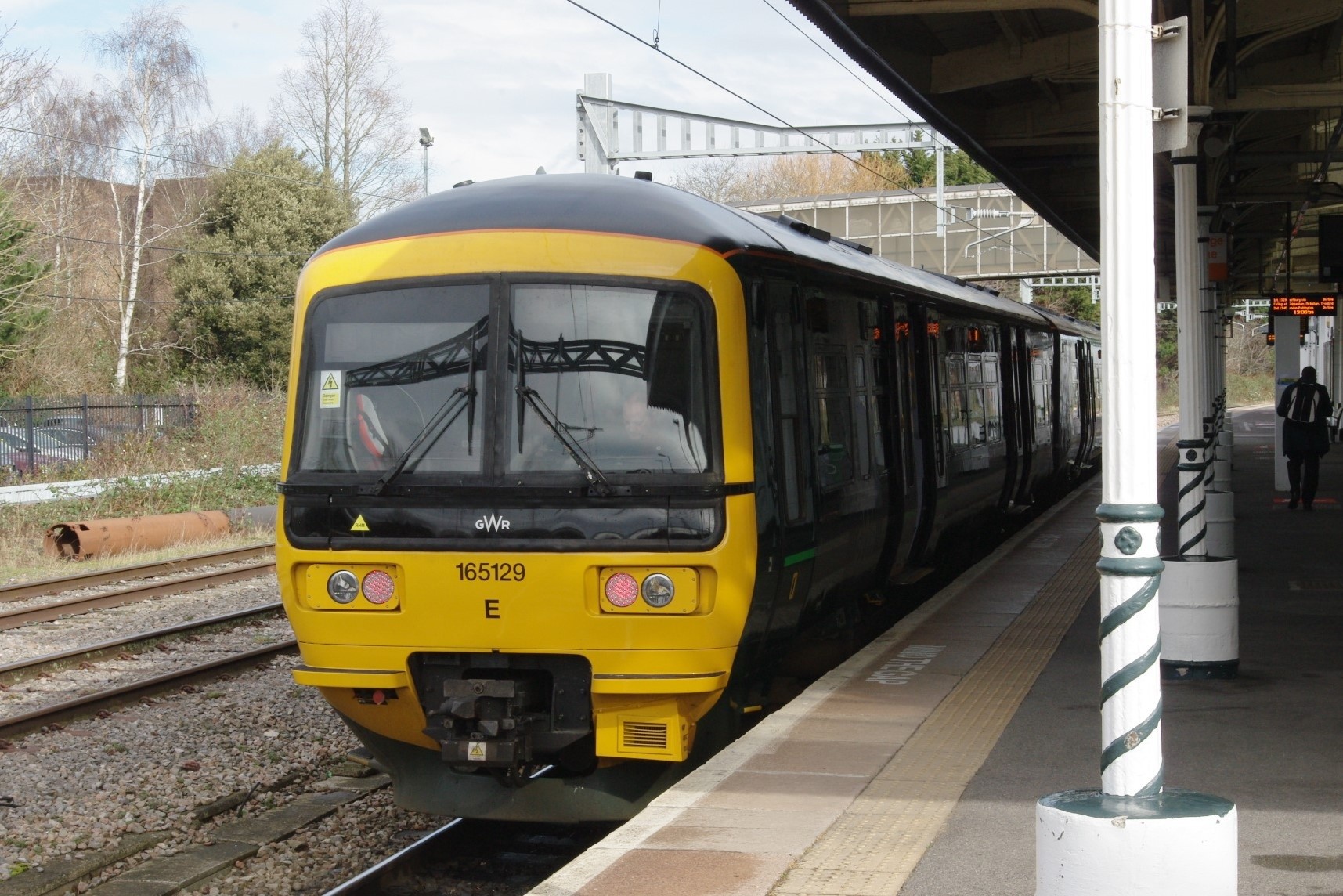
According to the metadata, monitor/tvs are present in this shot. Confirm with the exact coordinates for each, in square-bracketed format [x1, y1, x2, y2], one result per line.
[1267, 292, 1337, 346]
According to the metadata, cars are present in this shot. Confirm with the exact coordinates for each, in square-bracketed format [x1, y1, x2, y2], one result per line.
[0, 414, 167, 471]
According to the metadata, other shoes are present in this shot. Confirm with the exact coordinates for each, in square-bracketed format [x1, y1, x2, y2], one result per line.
[1302, 505, 1313, 512]
[1288, 500, 1297, 509]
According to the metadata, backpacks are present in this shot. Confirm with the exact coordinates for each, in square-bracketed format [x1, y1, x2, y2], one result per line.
[1287, 382, 1321, 423]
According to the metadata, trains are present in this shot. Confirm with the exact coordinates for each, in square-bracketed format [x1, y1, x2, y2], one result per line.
[274, 165, 1099, 823]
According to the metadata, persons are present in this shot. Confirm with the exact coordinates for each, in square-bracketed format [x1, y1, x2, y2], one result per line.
[1277, 365, 1333, 510]
[601, 391, 675, 454]
